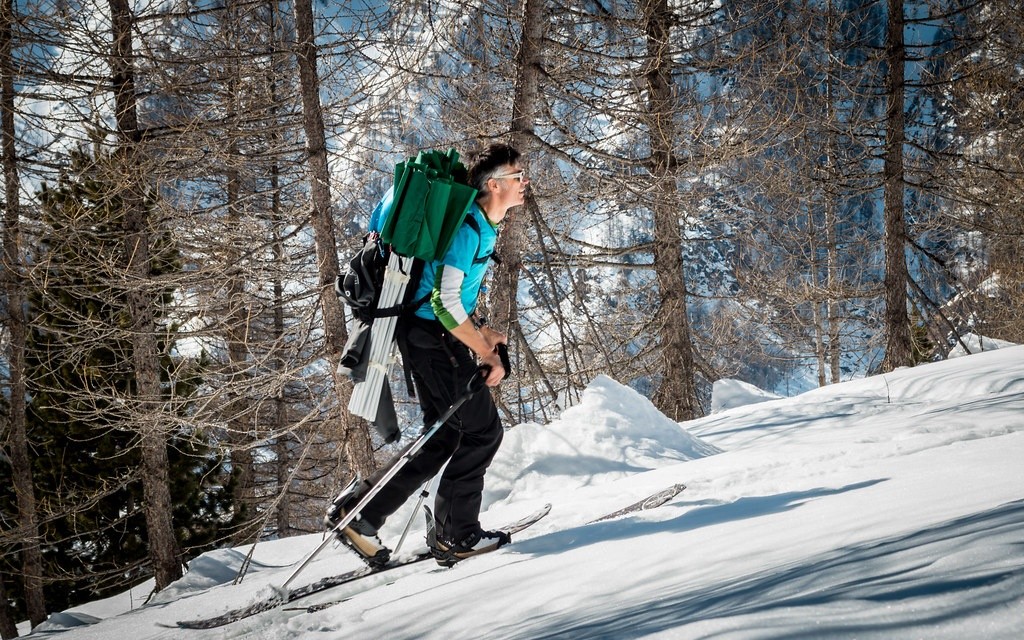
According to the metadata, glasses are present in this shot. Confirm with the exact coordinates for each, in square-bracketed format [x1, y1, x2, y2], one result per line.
[489, 168, 526, 183]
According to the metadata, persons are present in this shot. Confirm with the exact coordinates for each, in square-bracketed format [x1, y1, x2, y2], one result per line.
[322, 143, 529, 571]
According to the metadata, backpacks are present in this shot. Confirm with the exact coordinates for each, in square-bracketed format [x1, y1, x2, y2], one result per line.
[332, 214, 503, 396]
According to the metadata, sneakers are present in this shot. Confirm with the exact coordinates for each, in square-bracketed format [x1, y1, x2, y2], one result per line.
[432, 528, 508, 568]
[322, 503, 391, 565]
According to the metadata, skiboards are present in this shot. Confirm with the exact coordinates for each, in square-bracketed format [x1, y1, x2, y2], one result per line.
[177, 502, 553, 631]
